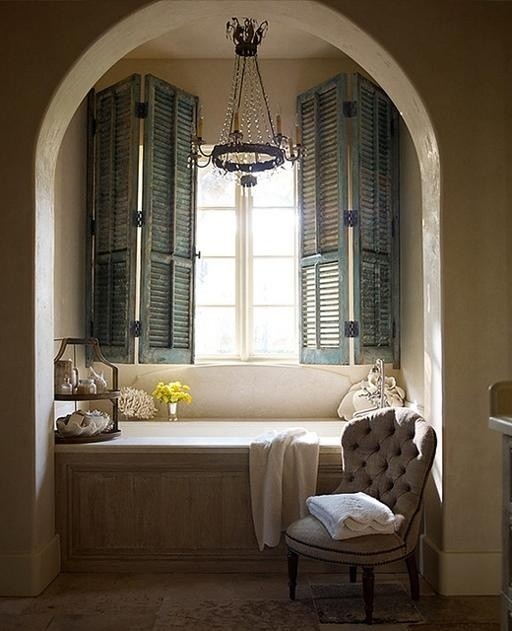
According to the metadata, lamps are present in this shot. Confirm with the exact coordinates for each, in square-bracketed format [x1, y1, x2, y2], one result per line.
[185, 17, 309, 189]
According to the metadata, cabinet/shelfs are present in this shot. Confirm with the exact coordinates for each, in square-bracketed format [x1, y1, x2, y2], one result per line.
[53, 338, 122, 444]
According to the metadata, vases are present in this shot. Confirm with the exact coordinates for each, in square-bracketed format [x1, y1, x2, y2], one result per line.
[167, 402, 179, 421]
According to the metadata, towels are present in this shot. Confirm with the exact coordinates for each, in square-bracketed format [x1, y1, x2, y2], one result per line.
[305, 492, 408, 542]
[249, 427, 321, 552]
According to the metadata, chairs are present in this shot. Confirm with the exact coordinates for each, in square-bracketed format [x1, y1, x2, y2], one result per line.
[283, 406, 437, 625]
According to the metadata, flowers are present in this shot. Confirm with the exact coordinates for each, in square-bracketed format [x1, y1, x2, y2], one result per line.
[152, 381, 191, 405]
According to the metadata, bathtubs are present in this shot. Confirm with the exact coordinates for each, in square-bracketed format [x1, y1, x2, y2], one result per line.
[108, 418, 347, 446]
